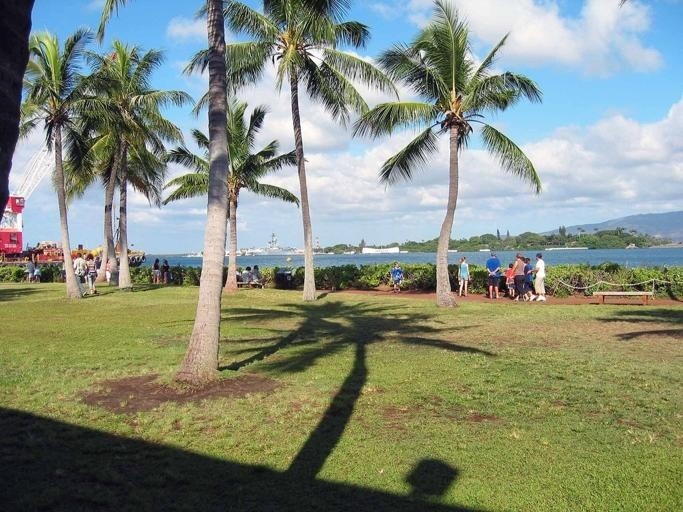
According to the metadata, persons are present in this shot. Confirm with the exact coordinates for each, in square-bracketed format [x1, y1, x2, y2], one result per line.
[151, 258, 162, 284]
[83, 253, 98, 299]
[486, 249, 547, 303]
[68, 250, 87, 299]
[161, 259, 169, 285]
[457, 255, 472, 298]
[22, 249, 113, 285]
[235, 265, 267, 289]
[390, 260, 405, 293]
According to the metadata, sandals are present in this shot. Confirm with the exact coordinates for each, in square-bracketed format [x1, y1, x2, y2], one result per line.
[489, 293, 518, 301]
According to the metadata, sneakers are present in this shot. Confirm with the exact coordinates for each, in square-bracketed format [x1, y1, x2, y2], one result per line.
[523, 295, 546, 302]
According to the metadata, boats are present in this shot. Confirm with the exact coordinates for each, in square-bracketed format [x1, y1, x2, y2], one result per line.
[21, 217, 146, 267]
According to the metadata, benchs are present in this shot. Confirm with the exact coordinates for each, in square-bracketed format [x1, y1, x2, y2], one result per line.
[237, 282, 264, 289]
[593, 291, 653, 306]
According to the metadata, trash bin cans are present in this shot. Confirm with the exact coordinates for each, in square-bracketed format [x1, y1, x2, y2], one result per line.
[275, 270, 294, 290]
